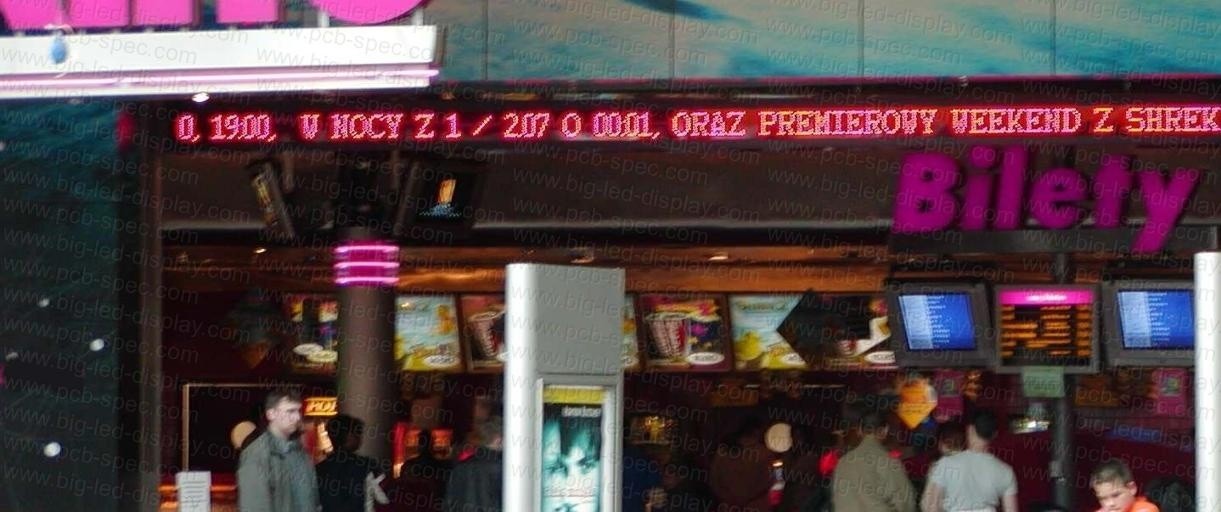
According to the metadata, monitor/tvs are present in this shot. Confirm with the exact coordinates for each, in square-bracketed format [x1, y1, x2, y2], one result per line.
[180, 382, 303, 471]
[1100, 281, 1195, 367]
[392, 160, 492, 246]
[884, 282, 995, 366]
[242, 160, 298, 240]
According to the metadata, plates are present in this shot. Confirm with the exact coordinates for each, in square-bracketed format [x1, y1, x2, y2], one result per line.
[686, 351, 725, 366]
[865, 348, 899, 369]
[294, 343, 338, 364]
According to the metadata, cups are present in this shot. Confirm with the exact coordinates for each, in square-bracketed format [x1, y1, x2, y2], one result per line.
[645, 309, 723, 358]
[465, 310, 501, 358]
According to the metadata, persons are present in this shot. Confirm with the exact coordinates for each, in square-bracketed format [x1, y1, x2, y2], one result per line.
[399, 428, 460, 512]
[618, 390, 1195, 512]
[237, 385, 324, 512]
[316, 415, 398, 512]
[444, 423, 504, 511]
